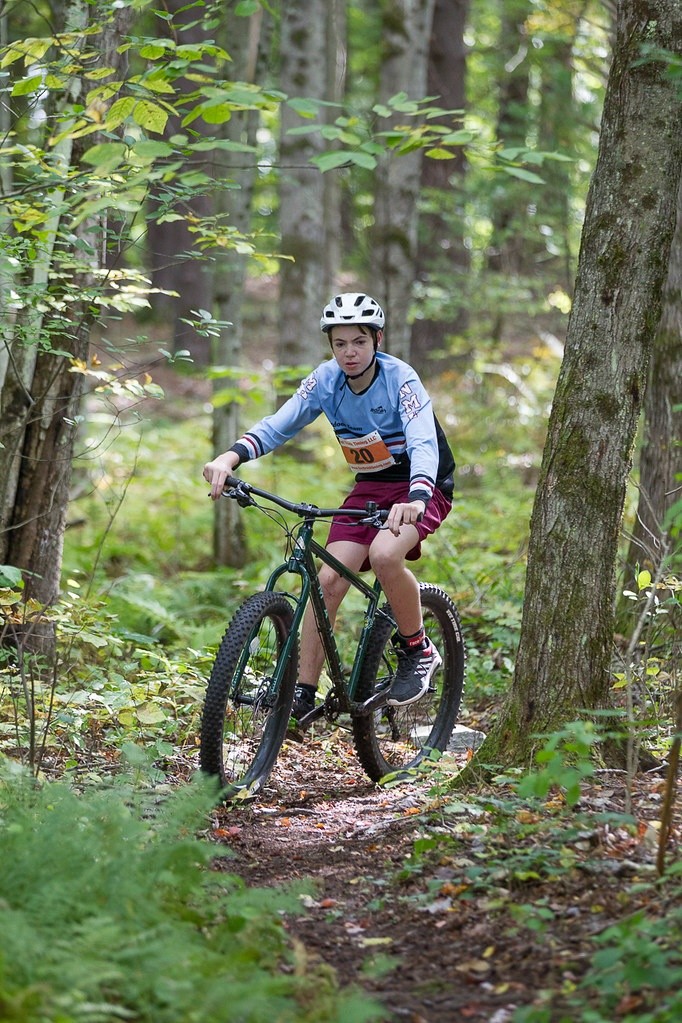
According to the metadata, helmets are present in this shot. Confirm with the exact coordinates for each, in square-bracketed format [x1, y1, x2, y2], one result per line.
[320, 291, 387, 327]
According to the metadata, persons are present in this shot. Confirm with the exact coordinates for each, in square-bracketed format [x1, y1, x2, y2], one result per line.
[204, 293, 456, 743]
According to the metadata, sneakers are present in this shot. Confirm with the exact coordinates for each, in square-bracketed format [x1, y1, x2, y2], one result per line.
[386, 636, 442, 704]
[290, 687, 315, 717]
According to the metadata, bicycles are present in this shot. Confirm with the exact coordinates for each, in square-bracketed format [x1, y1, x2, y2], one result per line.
[194, 464, 469, 806]
